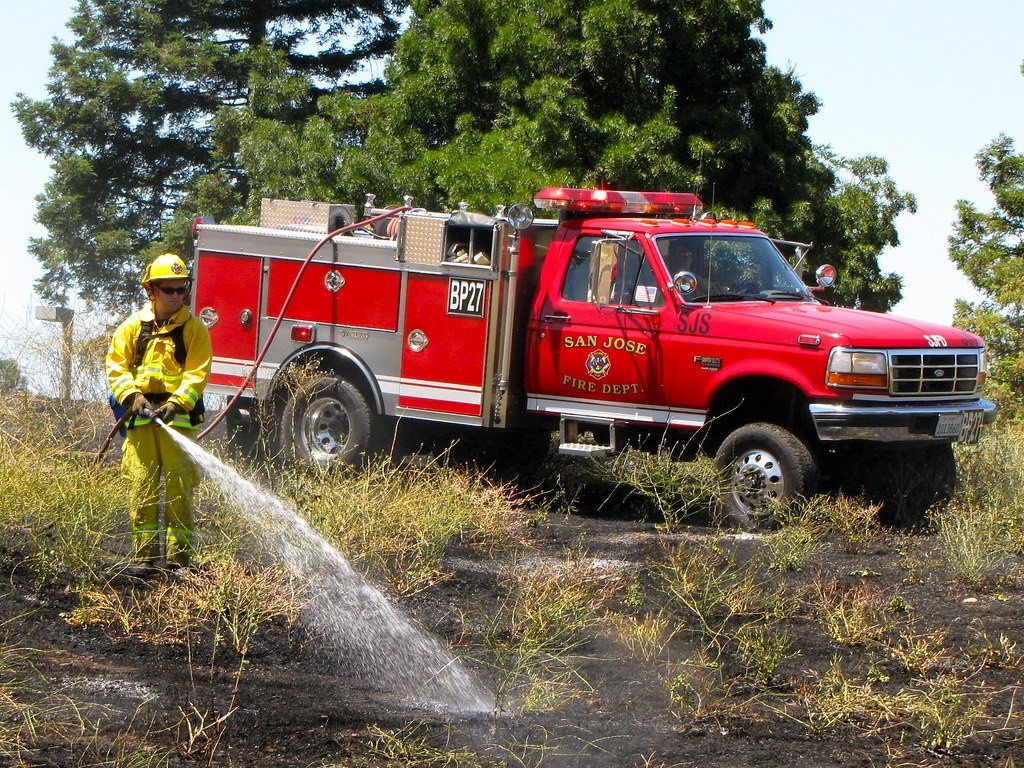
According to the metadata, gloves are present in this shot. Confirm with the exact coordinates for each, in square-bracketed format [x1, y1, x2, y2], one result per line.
[123, 392, 155, 418]
[153, 402, 181, 427]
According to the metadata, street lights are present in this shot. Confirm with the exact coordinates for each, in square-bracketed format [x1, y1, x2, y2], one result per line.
[33, 305, 75, 399]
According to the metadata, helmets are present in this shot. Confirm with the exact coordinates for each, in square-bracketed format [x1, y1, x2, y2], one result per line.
[140, 253, 188, 286]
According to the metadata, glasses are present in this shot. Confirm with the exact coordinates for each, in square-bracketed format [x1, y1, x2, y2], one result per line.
[150, 282, 189, 295]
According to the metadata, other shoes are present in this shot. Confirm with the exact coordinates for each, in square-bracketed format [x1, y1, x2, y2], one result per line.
[129, 560, 153, 577]
[160, 564, 180, 581]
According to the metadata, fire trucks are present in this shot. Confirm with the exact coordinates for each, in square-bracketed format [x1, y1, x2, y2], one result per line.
[183, 172, 997, 535]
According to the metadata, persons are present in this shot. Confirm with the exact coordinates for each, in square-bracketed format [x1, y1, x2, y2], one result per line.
[668, 241, 724, 300]
[106, 254, 212, 575]
